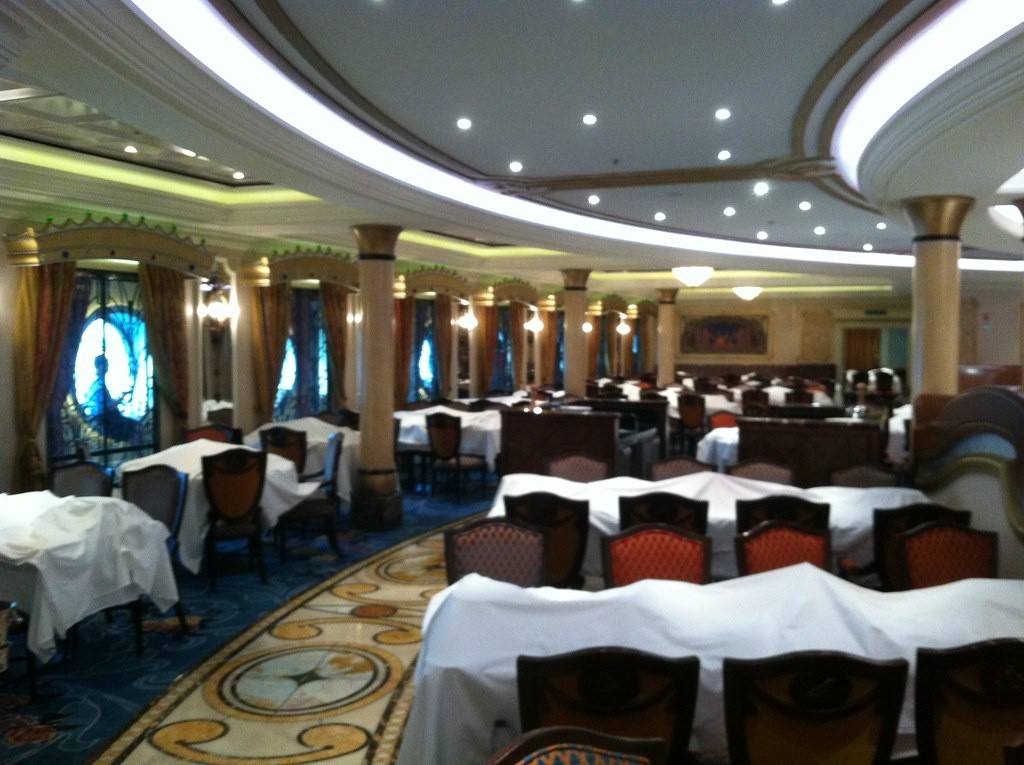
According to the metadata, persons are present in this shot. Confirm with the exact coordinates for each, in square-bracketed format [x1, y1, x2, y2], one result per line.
[847, 381, 884, 406]
[85, 356, 143, 446]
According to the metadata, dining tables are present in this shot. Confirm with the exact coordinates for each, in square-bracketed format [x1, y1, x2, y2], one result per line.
[243, 417, 360, 511]
[696, 426, 741, 476]
[396, 573, 1024, 765]
[120, 438, 321, 575]
[0, 488, 178, 674]
[761, 385, 834, 407]
[488, 472, 935, 574]
[392, 404, 503, 487]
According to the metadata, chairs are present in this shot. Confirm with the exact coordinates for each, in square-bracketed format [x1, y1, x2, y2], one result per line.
[694, 378, 716, 393]
[741, 390, 768, 411]
[678, 394, 710, 453]
[481, 639, 1024, 765]
[876, 371, 905, 406]
[444, 490, 1000, 594]
[707, 411, 739, 429]
[50, 461, 192, 655]
[425, 412, 490, 498]
[786, 388, 813, 411]
[546, 453, 611, 481]
[851, 369, 871, 405]
[827, 456, 907, 491]
[584, 377, 628, 400]
[723, 458, 798, 488]
[719, 372, 744, 388]
[186, 424, 345, 591]
[643, 455, 718, 486]
